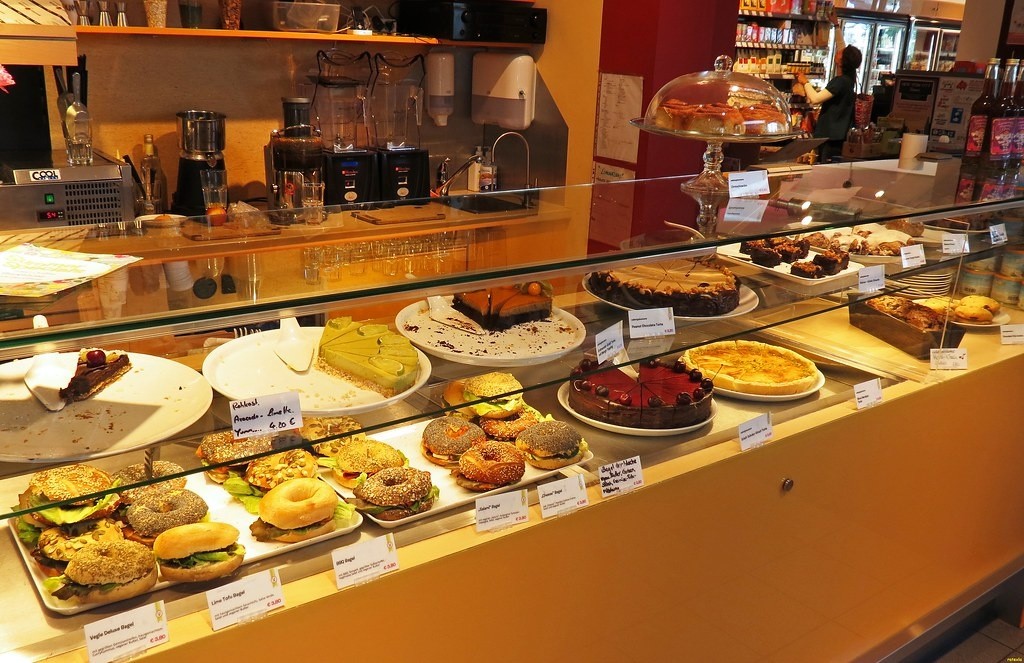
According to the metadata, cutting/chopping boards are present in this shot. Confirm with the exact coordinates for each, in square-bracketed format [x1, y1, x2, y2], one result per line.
[350, 204, 446, 224]
[182, 222, 283, 240]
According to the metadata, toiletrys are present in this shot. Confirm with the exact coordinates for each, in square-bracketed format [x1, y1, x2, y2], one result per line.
[468, 146, 497, 193]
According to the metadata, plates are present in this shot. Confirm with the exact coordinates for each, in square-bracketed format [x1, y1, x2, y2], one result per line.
[141, 217, 185, 228]
[1, 239, 143, 299]
[558, 220, 1012, 438]
[0, 295, 587, 617]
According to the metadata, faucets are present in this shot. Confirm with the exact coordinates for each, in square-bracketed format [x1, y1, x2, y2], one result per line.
[204, 151, 217, 167]
[491, 132, 538, 207]
[436, 155, 483, 196]
[305, 167, 317, 184]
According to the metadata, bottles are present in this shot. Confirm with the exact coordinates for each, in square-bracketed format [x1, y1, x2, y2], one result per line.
[868, 29, 897, 94]
[733, 0, 835, 162]
[140, 133, 163, 213]
[960, 57, 1024, 169]
[954, 160, 1024, 203]
[425, 54, 454, 125]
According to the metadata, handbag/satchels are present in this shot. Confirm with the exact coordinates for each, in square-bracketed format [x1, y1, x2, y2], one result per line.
[853, 75, 874, 128]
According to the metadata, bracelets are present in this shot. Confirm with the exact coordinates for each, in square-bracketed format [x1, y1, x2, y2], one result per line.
[834, 24, 839, 27]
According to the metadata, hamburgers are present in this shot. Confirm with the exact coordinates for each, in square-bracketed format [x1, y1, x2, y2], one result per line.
[11, 459, 245, 604]
[195, 416, 440, 545]
[421, 373, 589, 491]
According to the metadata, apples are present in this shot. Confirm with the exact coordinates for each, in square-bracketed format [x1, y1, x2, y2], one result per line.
[205, 207, 227, 225]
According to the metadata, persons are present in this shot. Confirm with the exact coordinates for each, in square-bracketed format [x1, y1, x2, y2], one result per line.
[797, 5, 862, 164]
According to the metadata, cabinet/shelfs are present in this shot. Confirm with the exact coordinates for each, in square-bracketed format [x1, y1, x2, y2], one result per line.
[731, 8, 831, 135]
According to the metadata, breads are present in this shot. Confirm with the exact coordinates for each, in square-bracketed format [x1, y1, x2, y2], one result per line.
[862, 295, 1001, 332]
[656, 98, 789, 135]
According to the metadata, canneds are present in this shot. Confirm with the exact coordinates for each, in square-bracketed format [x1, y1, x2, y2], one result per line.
[786, 62, 811, 75]
[957, 215, 1024, 312]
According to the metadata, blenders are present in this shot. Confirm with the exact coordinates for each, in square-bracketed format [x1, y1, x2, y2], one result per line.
[169, 110, 227, 222]
[307, 48, 378, 204]
[271, 94, 324, 223]
[364, 51, 430, 205]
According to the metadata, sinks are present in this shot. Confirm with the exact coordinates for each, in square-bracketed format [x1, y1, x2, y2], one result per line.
[429, 194, 535, 214]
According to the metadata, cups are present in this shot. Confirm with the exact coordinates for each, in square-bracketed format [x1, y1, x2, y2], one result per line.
[142, 0, 168, 28]
[178, 0, 202, 29]
[136, 199, 156, 216]
[61, 118, 93, 165]
[73, 0, 129, 26]
[218, 0, 242, 30]
[200, 170, 228, 210]
[897, 133, 927, 171]
[76, 228, 479, 319]
[301, 182, 323, 224]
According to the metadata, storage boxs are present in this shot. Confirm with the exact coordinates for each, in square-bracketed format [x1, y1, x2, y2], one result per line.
[770, 28, 776, 44]
[758, 27, 765, 43]
[776, 28, 782, 44]
[789, 29, 795, 44]
[783, 29, 789, 44]
[765, 27, 771, 43]
[740, 0, 802, 14]
[741, 24, 747, 41]
[736, 23, 742, 42]
[746, 26, 753, 42]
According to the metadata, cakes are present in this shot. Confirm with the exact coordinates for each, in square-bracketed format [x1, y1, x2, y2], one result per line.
[569, 356, 714, 430]
[318, 315, 422, 399]
[59, 349, 133, 401]
[589, 257, 740, 318]
[681, 340, 817, 396]
[451, 280, 554, 332]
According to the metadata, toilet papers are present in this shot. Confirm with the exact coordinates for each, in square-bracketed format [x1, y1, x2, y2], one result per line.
[899, 132, 929, 159]
[898, 159, 924, 170]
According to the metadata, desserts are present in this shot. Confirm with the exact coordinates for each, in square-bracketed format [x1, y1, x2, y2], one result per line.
[739, 220, 925, 281]
